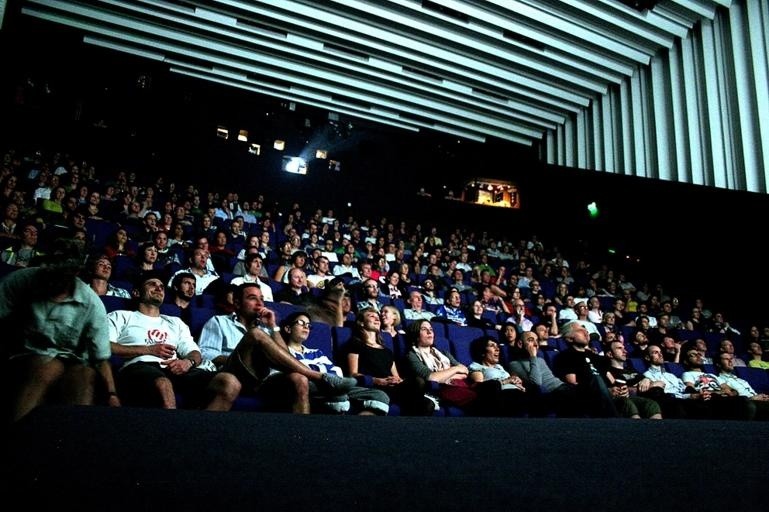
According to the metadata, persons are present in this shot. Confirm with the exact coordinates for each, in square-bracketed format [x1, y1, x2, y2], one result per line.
[1, 142, 769, 420]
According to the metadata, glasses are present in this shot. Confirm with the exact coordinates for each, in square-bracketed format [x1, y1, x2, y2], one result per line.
[290, 320, 313, 329]
[689, 352, 702, 357]
[580, 305, 588, 310]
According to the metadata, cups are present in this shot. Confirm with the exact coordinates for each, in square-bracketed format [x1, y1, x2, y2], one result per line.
[615, 379, 626, 399]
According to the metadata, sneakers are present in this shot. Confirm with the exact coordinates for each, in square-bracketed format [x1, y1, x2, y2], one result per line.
[316, 372, 357, 397]
